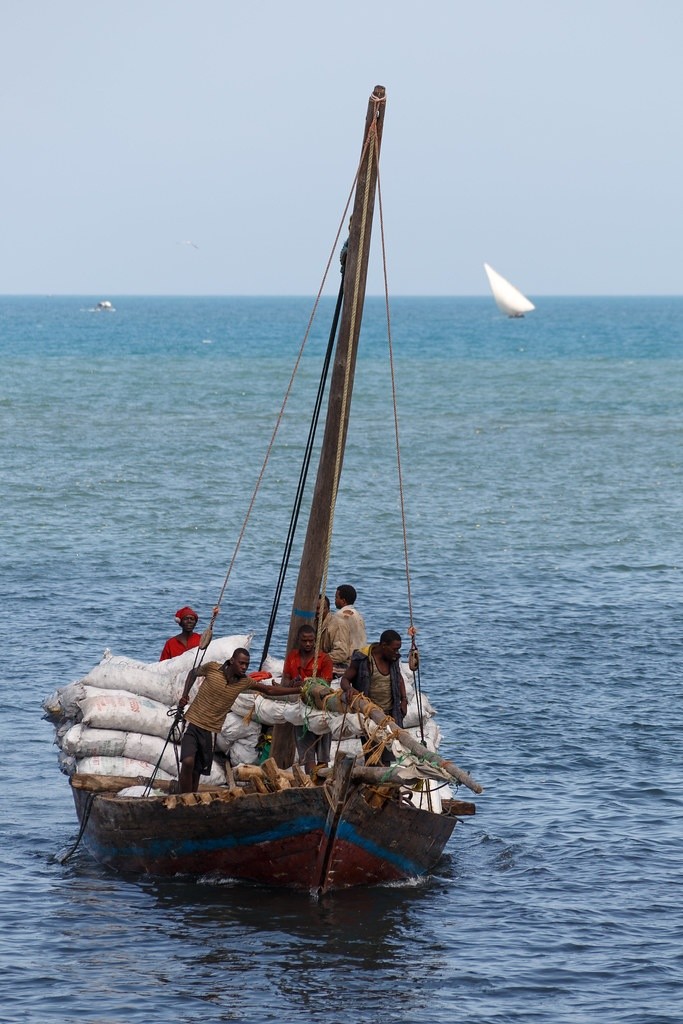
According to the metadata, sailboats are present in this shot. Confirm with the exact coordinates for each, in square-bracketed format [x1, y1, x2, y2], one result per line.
[69, 86, 484, 909]
[483, 262, 536, 319]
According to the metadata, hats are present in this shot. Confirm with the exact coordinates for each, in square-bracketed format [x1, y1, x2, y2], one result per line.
[174, 607, 198, 624]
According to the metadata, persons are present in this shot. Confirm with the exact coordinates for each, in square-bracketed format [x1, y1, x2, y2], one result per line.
[339, 630, 408, 767]
[317, 593, 348, 664]
[334, 584, 368, 668]
[160, 606, 202, 662]
[178, 648, 306, 793]
[281, 625, 333, 774]
